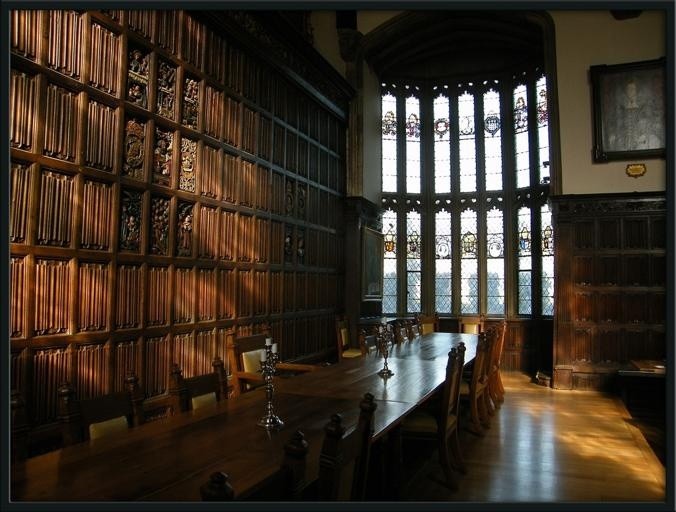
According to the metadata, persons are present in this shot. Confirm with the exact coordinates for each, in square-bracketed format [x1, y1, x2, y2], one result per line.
[607, 78, 657, 151]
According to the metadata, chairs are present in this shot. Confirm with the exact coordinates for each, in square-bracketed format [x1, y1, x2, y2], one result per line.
[11, 309, 510, 502]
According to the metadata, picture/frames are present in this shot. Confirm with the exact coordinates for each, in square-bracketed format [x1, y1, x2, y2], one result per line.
[589, 56, 667, 164]
